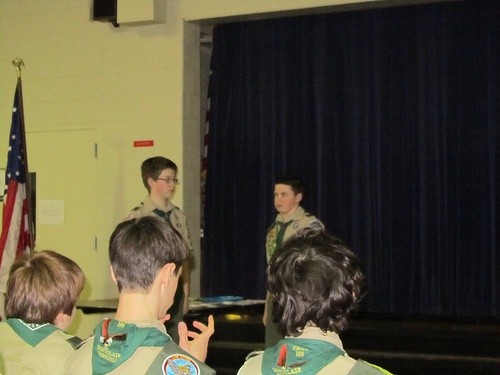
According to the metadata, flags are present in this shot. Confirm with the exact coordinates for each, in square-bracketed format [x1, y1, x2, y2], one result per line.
[0, 76, 35, 295]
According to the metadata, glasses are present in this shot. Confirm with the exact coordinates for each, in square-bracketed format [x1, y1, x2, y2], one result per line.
[156, 177, 178, 185]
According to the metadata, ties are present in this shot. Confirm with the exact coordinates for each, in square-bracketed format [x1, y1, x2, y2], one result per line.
[273, 220, 293, 250]
[153, 208, 172, 227]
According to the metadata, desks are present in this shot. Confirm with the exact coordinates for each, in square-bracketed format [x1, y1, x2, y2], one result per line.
[74, 298, 266, 318]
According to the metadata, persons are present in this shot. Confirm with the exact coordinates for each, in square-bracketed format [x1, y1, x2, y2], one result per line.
[234, 226, 395, 375]
[64, 215, 217, 375]
[122, 155, 195, 347]
[0, 250, 86, 375]
[262, 173, 326, 350]
